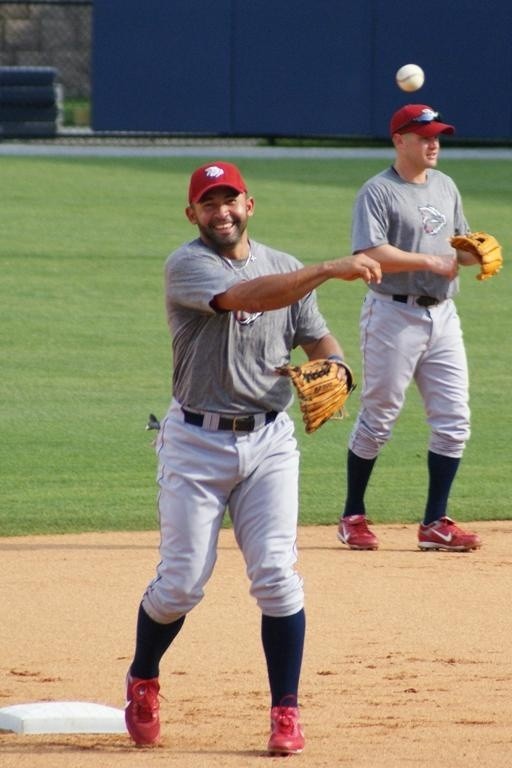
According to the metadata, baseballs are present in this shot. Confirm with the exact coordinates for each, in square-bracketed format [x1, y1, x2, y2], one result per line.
[396, 64, 424, 92]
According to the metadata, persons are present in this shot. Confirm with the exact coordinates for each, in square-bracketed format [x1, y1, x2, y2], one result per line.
[122, 166, 383, 758]
[336, 103, 484, 553]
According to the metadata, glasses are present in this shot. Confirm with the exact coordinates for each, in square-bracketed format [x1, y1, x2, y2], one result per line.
[397, 112, 439, 131]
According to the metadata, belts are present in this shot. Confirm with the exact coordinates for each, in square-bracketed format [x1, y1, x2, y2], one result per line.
[394, 295, 438, 307]
[181, 405, 278, 431]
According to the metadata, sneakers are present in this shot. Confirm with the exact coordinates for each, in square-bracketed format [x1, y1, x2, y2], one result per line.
[267, 706, 305, 755]
[417, 516, 482, 550]
[337, 514, 379, 550]
[125, 670, 161, 744]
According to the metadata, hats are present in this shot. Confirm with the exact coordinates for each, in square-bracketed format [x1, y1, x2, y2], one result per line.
[189, 161, 247, 206]
[389, 105, 454, 138]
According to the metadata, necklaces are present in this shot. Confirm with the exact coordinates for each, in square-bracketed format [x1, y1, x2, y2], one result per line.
[231, 249, 258, 272]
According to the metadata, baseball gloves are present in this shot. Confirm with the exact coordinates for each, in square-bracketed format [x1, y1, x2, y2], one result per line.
[275, 360, 355, 435]
[448, 232, 503, 279]
